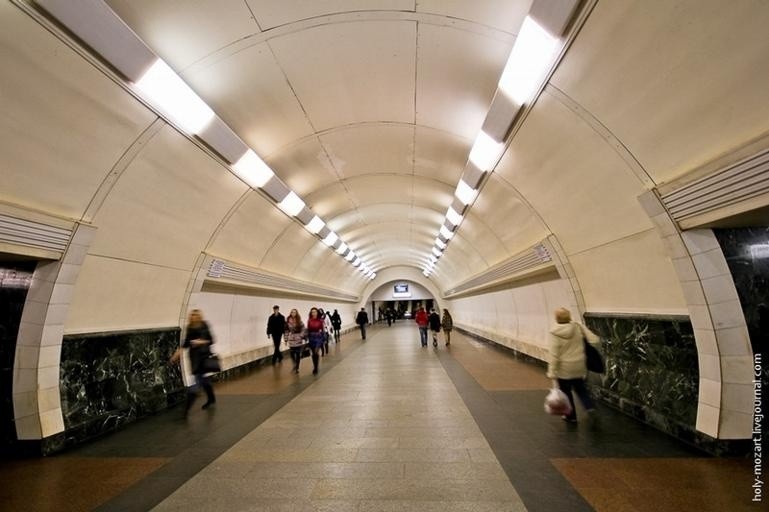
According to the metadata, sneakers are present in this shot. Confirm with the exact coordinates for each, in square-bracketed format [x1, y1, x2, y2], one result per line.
[584, 402, 599, 412]
[561, 415, 577, 423]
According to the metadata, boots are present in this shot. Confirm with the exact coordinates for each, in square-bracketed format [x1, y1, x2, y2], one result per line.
[271, 343, 329, 374]
[182, 393, 198, 420]
[202, 384, 216, 409]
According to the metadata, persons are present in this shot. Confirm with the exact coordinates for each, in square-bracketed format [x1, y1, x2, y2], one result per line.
[385, 308, 397, 327]
[415, 307, 453, 348]
[166, 309, 216, 416]
[266, 304, 342, 375]
[355, 307, 368, 339]
[546, 307, 603, 422]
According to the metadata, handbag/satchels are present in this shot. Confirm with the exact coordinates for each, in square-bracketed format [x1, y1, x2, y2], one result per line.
[191, 353, 221, 374]
[585, 341, 604, 373]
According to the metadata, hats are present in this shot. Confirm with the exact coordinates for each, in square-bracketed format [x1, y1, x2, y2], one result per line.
[273, 305, 280, 310]
